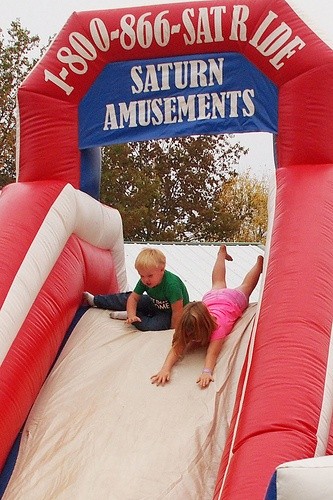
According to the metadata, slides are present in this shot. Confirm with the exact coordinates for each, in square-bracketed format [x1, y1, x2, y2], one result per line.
[1, 177, 333, 496]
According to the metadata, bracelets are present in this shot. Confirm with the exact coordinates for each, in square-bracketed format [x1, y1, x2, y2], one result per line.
[203, 368, 212, 373]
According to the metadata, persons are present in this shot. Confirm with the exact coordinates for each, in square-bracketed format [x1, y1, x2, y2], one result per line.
[86, 249, 189, 331]
[150, 245, 265, 389]
